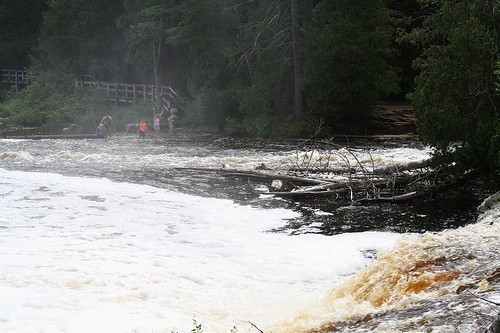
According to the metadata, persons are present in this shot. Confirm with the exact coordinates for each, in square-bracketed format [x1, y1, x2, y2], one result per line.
[94, 105, 178, 142]
